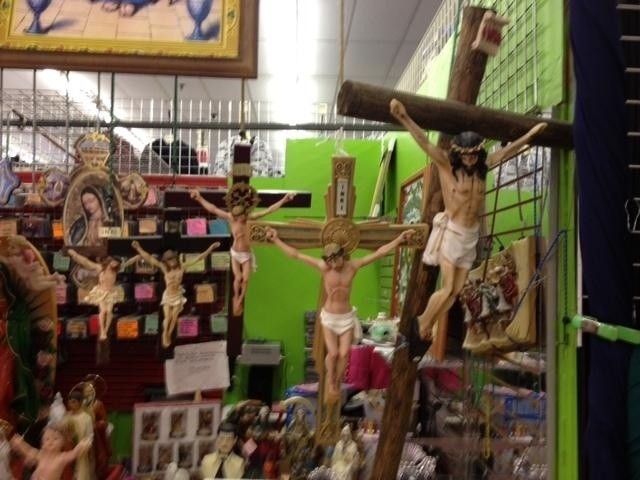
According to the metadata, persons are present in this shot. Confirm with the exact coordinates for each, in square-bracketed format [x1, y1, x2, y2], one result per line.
[66, 246, 144, 345]
[132, 238, 222, 350]
[263, 222, 414, 412]
[388, 98, 550, 365]
[186, 184, 299, 319]
[73, 183, 112, 249]
[0, 382, 113, 479]
[190, 397, 360, 478]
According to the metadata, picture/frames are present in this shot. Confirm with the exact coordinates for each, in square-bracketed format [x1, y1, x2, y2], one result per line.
[390, 162, 432, 321]
[0, 0, 261, 78]
